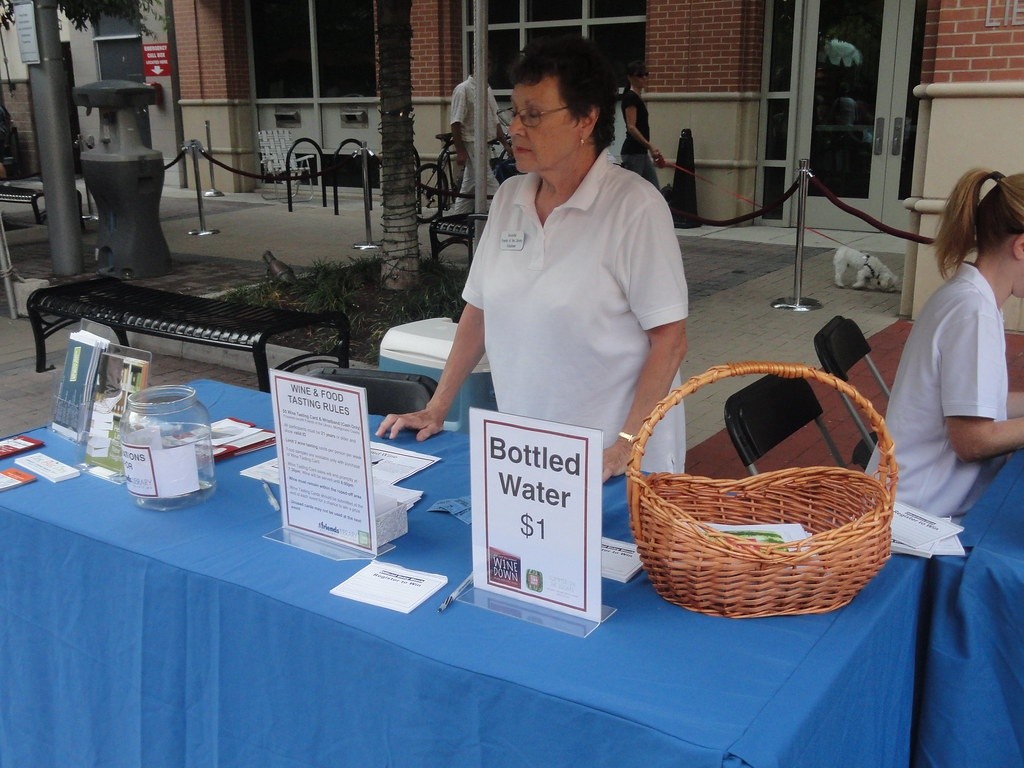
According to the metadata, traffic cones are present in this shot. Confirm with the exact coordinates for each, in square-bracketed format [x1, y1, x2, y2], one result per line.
[667, 128, 704, 230]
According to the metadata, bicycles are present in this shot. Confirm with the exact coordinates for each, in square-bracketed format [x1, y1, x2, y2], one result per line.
[411, 132, 513, 224]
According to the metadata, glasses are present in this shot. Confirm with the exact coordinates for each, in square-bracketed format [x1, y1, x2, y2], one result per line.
[496, 106, 568, 128]
[633, 72, 650, 78]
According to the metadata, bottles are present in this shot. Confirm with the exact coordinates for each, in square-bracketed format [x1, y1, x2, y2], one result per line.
[263, 250, 296, 283]
[118, 385, 217, 513]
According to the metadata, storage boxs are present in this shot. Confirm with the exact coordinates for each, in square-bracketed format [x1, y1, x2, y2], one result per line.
[378, 315, 497, 436]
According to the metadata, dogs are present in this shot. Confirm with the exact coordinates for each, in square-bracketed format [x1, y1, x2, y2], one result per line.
[833, 245, 898, 293]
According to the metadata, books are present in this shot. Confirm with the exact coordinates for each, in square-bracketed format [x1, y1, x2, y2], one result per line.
[51, 330, 111, 442]
[891, 501, 966, 558]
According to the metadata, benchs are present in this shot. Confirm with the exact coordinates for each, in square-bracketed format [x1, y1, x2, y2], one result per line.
[27, 277, 350, 392]
[430, 209, 490, 267]
[0, 183, 88, 226]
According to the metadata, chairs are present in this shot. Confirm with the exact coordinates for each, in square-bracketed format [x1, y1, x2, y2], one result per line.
[726, 371, 850, 479]
[814, 313, 891, 464]
[258, 128, 312, 203]
[307, 368, 441, 420]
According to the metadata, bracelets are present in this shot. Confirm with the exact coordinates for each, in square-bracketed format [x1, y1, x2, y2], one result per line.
[618, 432, 634, 441]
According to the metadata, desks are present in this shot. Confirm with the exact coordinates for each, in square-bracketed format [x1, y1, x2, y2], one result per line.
[0, 378, 926, 768]
[910, 447, 1024, 768]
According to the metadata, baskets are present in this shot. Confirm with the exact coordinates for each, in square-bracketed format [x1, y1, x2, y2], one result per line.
[627, 361, 897, 619]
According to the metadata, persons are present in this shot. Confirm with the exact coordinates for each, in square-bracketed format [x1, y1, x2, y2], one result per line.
[0, 108, 10, 179]
[865, 168, 1024, 525]
[812, 68, 873, 195]
[450, 51, 514, 214]
[375, 32, 689, 483]
[620, 61, 665, 189]
